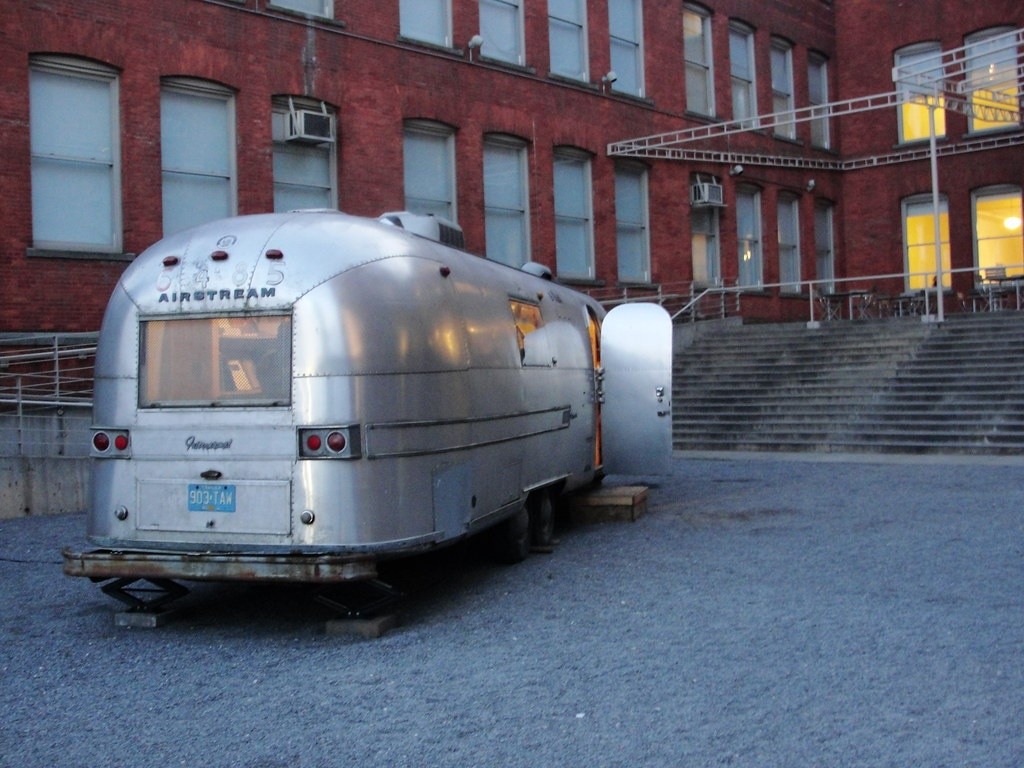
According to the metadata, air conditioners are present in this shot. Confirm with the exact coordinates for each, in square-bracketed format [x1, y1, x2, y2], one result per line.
[690, 182, 723, 207]
[284, 108, 331, 147]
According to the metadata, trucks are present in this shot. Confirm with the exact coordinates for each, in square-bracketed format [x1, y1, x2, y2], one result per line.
[61, 204, 675, 618]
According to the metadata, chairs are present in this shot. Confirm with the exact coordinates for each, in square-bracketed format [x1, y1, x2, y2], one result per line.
[816, 268, 1024, 315]
